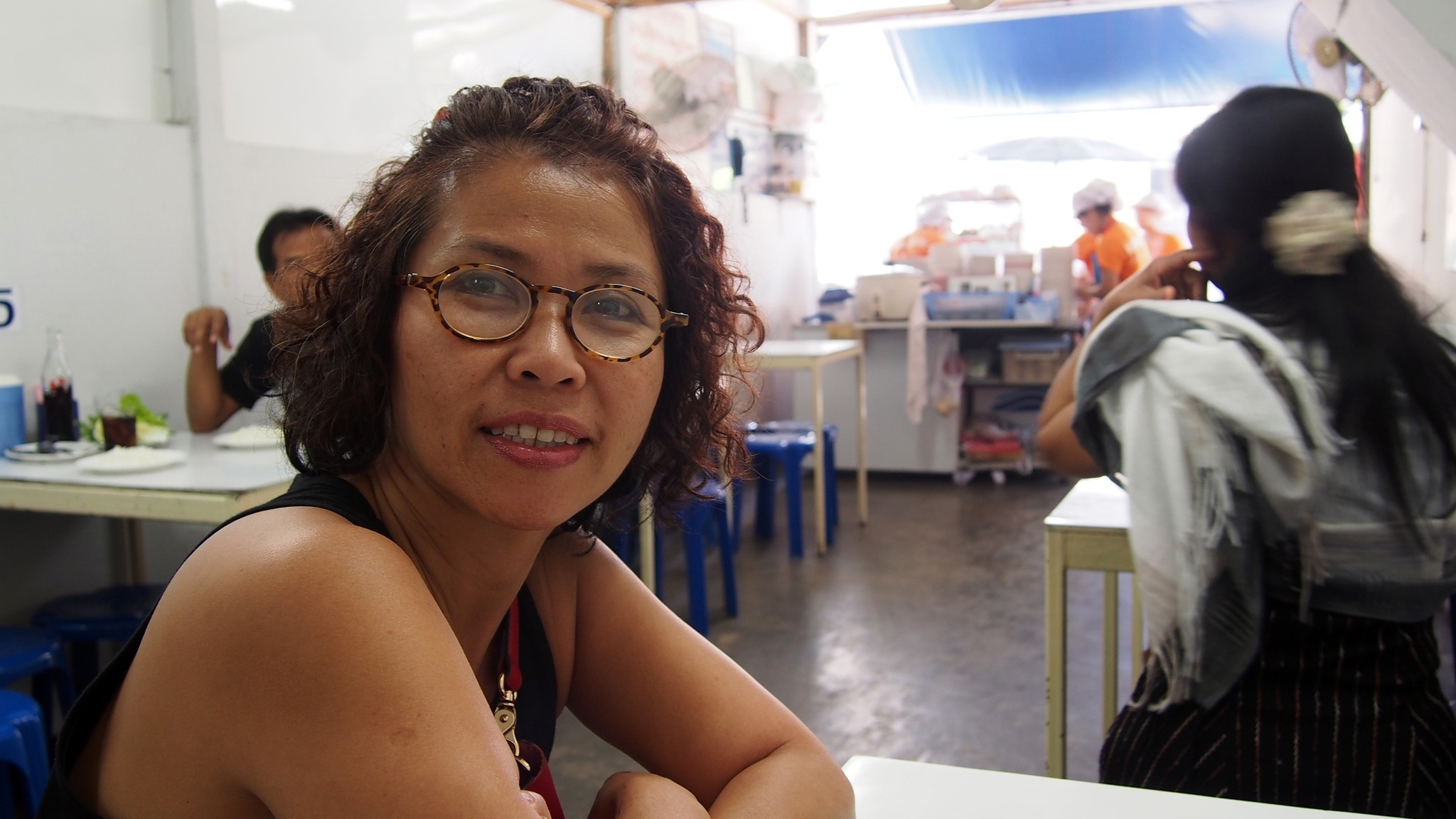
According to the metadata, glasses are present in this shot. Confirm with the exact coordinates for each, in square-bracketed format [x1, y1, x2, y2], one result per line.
[398, 263, 689, 364]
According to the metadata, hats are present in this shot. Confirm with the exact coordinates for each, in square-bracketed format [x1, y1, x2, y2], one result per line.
[1073, 181, 1115, 217]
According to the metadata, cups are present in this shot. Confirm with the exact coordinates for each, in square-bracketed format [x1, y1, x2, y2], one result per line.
[101, 417, 135, 451]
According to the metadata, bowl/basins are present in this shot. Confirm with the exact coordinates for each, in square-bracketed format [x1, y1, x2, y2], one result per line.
[947, 248, 1074, 319]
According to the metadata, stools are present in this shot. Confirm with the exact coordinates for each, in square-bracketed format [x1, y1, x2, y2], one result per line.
[0, 691, 51, 815]
[34, 583, 168, 711]
[0, 627, 72, 722]
[615, 482, 739, 636]
[744, 419, 839, 557]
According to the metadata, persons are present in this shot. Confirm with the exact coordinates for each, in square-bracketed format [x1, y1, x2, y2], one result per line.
[1035, 86, 1456, 819]
[183, 208, 341, 433]
[889, 207, 952, 258]
[52, 76, 855, 819]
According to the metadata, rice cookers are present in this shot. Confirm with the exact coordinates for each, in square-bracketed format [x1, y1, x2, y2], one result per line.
[856, 264, 919, 320]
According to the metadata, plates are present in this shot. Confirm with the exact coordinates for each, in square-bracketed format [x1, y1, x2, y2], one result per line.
[212, 429, 285, 448]
[77, 447, 186, 472]
[3, 441, 97, 460]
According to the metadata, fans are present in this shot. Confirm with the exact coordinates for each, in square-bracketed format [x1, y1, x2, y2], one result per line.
[1288, 4, 1386, 244]
[644, 53, 736, 151]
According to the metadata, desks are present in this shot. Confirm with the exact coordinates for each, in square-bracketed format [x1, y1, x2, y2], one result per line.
[0, 432, 290, 583]
[721, 340, 868, 556]
[1043, 478, 1143, 779]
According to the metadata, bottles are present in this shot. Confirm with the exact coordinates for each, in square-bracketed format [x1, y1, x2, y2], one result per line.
[42, 330, 76, 442]
[0, 374, 28, 456]
